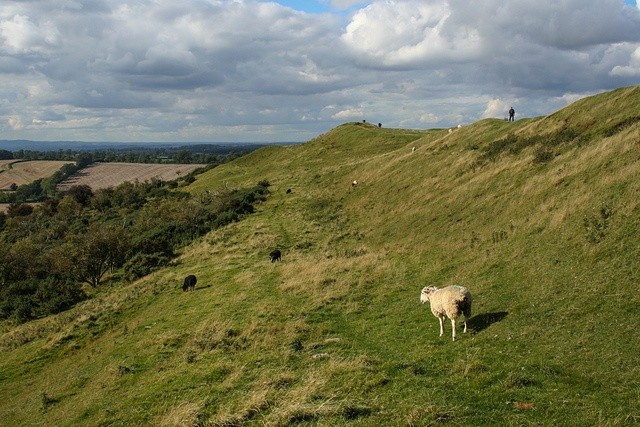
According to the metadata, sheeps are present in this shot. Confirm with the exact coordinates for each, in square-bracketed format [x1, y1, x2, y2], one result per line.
[182, 275, 197, 292]
[420, 284, 472, 342]
[269, 249, 281, 263]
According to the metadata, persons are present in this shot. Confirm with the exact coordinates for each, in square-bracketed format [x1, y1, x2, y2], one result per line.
[508, 106, 515, 121]
